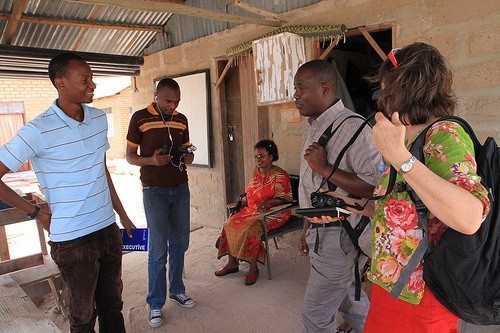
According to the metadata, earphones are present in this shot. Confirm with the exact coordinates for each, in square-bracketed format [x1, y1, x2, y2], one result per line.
[155, 95, 157, 102]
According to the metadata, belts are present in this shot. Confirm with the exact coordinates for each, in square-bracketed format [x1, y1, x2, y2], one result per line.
[311, 221, 343, 229]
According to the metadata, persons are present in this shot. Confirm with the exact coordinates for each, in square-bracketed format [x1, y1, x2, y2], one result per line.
[0, 51, 137, 333]
[310, 42, 491, 333]
[126, 78, 196, 327]
[293, 60, 386, 333]
[214, 140, 292, 283]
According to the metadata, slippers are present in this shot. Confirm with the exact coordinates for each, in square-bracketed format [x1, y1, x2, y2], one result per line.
[244, 270, 259, 286]
[215, 267, 239, 276]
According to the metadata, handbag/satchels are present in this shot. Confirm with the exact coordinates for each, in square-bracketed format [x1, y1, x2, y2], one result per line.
[406, 116, 500, 326]
[318, 112, 375, 259]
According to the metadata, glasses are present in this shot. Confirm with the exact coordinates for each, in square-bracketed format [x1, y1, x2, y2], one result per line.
[254, 153, 270, 160]
[387, 47, 401, 69]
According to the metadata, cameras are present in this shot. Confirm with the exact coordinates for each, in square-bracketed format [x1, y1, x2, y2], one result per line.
[310, 192, 347, 209]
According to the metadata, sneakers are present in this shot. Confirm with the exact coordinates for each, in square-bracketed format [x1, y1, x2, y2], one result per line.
[147, 305, 163, 328]
[168, 293, 195, 308]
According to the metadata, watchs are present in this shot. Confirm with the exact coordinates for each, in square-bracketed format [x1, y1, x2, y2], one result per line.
[26, 206, 40, 219]
[398, 156, 417, 175]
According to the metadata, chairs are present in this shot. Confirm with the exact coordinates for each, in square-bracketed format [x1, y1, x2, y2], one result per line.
[225, 173, 304, 280]
